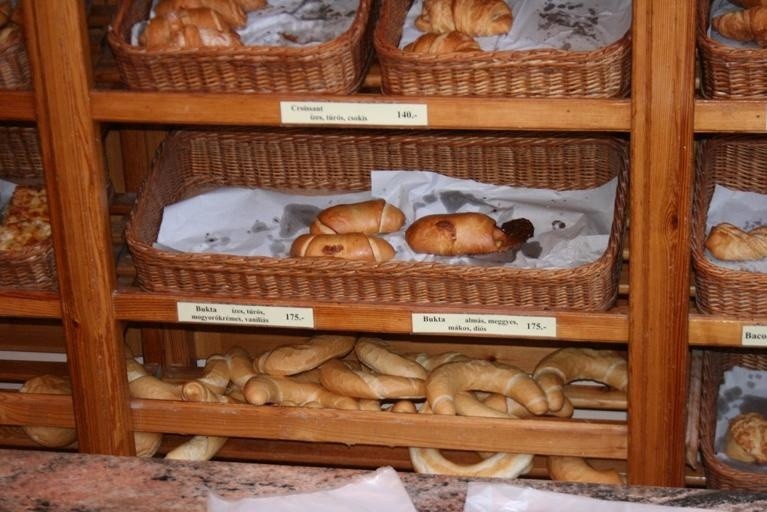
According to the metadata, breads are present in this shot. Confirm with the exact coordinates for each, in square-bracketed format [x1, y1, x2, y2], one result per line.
[720, 411, 766, 468]
[288, 233, 396, 264]
[121, 334, 629, 488]
[310, 196, 406, 238]
[402, 30, 478, 55]
[712, 0, 767, 50]
[405, 212, 506, 256]
[704, 219, 767, 262]
[139, 1, 267, 49]
[416, 0, 513, 35]
[16, 374, 77, 449]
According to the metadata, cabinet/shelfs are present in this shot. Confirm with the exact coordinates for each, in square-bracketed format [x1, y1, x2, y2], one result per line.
[0, 0, 767, 490]
